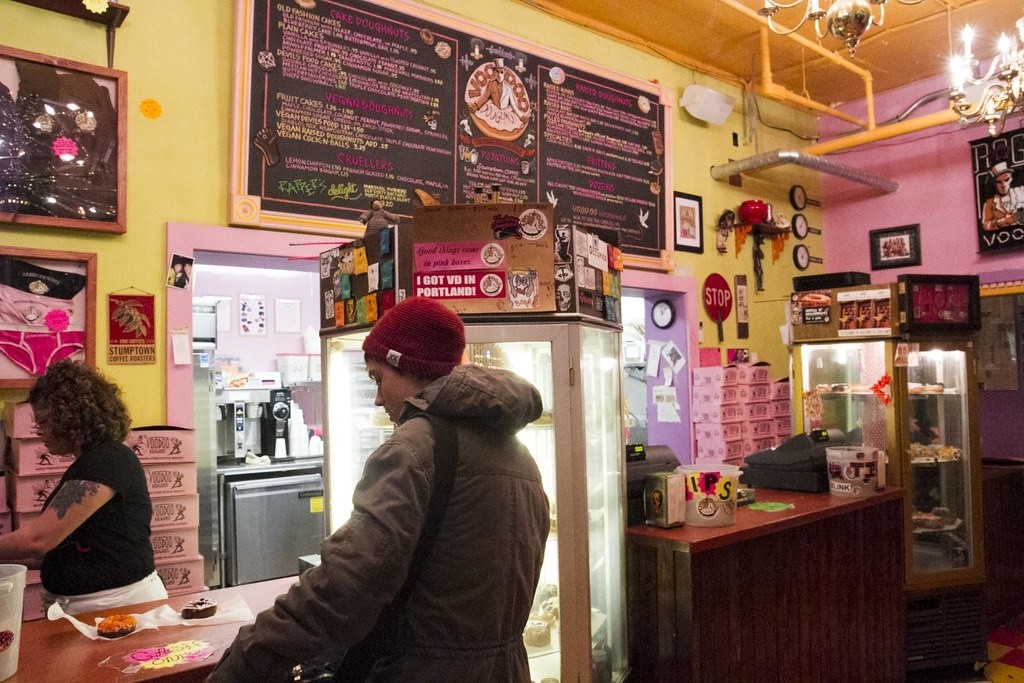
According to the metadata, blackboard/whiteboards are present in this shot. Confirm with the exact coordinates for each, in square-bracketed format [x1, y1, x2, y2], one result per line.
[226, 1, 676, 272]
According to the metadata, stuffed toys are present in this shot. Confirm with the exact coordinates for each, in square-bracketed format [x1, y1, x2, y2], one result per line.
[360, 197, 396, 236]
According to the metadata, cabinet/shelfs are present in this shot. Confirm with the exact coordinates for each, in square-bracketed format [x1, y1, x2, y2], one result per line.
[319, 317, 621, 682]
[789, 338, 984, 590]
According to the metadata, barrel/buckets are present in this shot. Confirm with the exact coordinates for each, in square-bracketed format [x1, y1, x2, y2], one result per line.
[677, 464, 738, 526]
[309, 353, 321, 381]
[825, 445, 878, 498]
[276, 353, 309, 386]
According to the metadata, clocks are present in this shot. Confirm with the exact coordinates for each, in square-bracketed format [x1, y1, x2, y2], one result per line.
[790, 185, 806, 210]
[792, 244, 810, 270]
[791, 214, 809, 239]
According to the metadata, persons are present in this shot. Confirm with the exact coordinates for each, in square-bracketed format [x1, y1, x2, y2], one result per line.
[168, 262, 192, 287]
[0, 358, 168, 615]
[205, 297, 550, 682]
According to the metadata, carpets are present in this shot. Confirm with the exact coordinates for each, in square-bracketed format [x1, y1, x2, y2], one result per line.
[984, 626, 1024, 683]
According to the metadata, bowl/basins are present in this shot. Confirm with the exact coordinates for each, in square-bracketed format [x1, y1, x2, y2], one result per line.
[739, 200, 767, 222]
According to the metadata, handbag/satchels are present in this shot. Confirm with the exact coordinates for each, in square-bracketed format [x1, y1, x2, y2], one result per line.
[284, 661, 335, 683]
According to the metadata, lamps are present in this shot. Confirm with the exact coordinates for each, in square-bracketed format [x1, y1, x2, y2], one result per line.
[944, 16, 1024, 138]
[757, 0, 922, 58]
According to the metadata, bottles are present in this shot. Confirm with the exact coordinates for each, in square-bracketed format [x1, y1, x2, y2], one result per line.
[464, 147, 470, 162]
[485, 194, 492, 204]
[473, 186, 484, 204]
[459, 145, 465, 160]
[471, 148, 478, 164]
[491, 184, 501, 203]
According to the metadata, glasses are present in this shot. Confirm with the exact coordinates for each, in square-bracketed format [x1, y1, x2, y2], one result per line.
[31, 415, 51, 429]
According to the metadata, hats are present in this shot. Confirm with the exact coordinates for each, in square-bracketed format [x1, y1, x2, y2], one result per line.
[363, 297, 466, 381]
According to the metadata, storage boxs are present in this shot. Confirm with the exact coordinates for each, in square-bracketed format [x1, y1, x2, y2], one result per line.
[1, 400, 211, 622]
[691, 359, 792, 470]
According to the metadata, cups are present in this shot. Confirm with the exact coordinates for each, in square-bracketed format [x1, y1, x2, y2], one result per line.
[521, 161, 529, 174]
[274, 438, 288, 458]
[288, 400, 323, 459]
[0, 564, 28, 682]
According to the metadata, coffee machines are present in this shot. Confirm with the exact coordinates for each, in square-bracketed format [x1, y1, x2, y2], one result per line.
[216, 367, 295, 465]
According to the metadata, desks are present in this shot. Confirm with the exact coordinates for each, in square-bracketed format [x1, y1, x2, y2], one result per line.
[0, 571, 298, 683]
[626, 482, 906, 683]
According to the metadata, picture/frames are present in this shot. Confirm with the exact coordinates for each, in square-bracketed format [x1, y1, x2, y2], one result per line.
[869, 222, 922, 271]
[1, 43, 128, 233]
[672, 191, 704, 253]
[0, 246, 98, 388]
